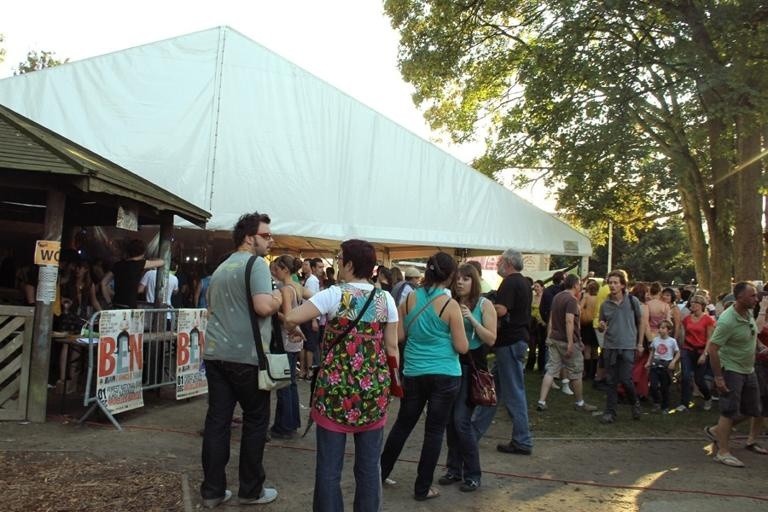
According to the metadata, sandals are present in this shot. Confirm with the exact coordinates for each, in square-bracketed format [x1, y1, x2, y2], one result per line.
[419, 485, 441, 500]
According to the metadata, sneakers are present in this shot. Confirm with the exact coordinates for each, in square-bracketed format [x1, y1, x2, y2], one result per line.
[598, 410, 618, 424]
[703, 397, 713, 412]
[702, 424, 721, 448]
[382, 477, 398, 487]
[574, 401, 598, 413]
[675, 404, 689, 412]
[239, 485, 279, 505]
[496, 441, 533, 456]
[551, 381, 560, 390]
[631, 405, 642, 419]
[459, 479, 482, 492]
[662, 408, 669, 415]
[650, 403, 662, 412]
[437, 470, 463, 486]
[536, 402, 549, 410]
[201, 488, 234, 509]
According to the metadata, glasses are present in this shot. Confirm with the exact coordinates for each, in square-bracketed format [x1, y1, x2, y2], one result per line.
[249, 231, 274, 241]
[333, 255, 345, 262]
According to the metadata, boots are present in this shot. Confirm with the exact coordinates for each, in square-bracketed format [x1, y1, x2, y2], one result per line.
[561, 378, 574, 396]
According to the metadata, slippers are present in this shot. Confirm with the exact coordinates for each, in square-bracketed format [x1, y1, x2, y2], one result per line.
[744, 442, 768, 457]
[229, 413, 299, 439]
[713, 448, 745, 471]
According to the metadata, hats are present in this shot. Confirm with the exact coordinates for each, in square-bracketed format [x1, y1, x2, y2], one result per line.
[404, 267, 422, 278]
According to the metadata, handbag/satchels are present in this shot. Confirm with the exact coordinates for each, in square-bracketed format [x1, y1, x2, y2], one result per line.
[308, 364, 322, 409]
[636, 330, 650, 354]
[255, 352, 294, 392]
[469, 367, 499, 408]
